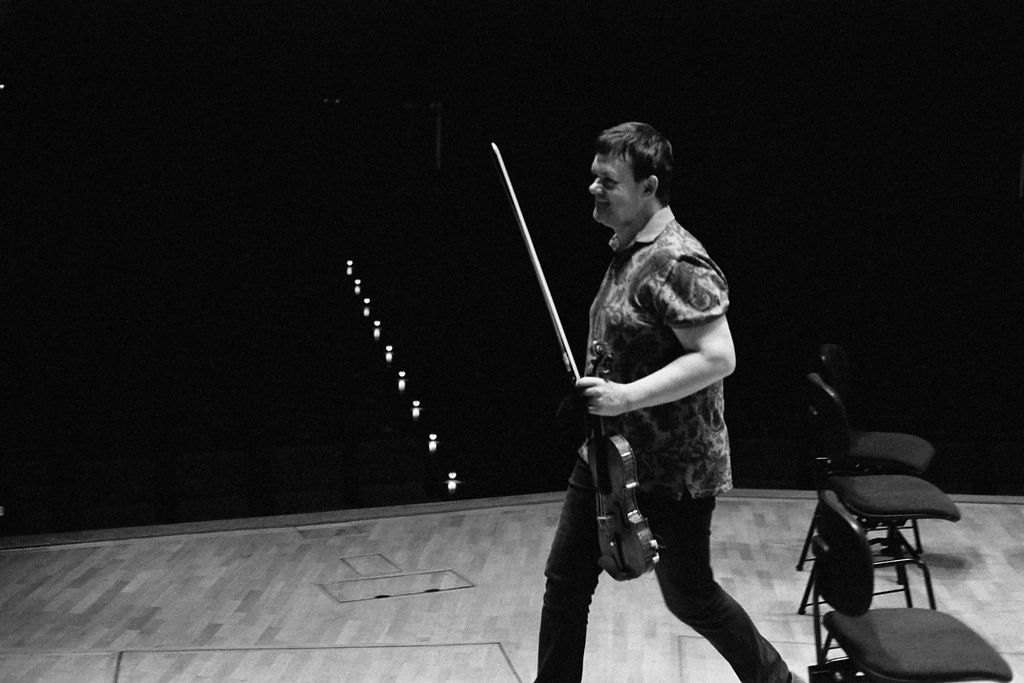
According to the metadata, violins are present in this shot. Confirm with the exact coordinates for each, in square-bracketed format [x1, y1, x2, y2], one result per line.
[583, 338, 659, 581]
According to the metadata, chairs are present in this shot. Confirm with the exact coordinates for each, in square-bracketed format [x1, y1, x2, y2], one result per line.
[795, 343, 962, 615]
[1, 436, 430, 539]
[807, 491, 1013, 683]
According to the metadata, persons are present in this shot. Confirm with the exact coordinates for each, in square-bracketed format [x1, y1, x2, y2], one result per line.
[534, 120, 794, 683]
